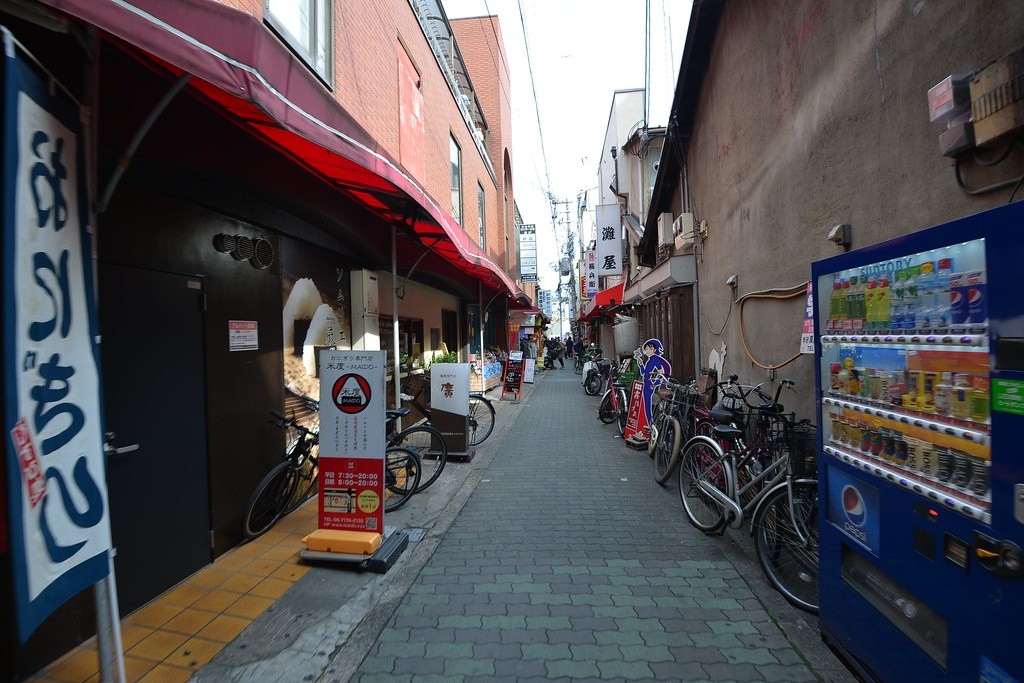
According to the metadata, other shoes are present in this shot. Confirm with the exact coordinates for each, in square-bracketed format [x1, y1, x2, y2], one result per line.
[560, 366, 565, 369]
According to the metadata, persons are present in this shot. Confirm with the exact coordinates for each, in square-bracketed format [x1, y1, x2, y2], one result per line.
[542, 335, 583, 370]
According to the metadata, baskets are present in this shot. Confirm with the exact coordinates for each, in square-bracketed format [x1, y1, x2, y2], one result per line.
[392, 378, 424, 402]
[678, 387, 818, 474]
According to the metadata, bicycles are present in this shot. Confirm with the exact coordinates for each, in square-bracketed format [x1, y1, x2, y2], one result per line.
[390, 367, 496, 447]
[598, 362, 819, 617]
[241, 394, 447, 540]
[573, 353, 579, 373]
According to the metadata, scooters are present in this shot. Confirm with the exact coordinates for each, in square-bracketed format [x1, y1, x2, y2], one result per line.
[582, 341, 608, 394]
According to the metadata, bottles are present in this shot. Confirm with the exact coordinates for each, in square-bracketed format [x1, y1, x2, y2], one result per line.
[830, 418, 988, 497]
[829, 259, 952, 327]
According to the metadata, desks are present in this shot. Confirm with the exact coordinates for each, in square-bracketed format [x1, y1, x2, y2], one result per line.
[470, 365, 503, 395]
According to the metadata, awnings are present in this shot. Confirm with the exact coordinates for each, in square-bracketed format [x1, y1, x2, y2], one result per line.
[46, 1, 534, 314]
[585, 283, 624, 318]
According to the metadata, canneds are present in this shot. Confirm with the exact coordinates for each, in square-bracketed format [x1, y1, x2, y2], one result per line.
[831, 418, 908, 466]
[829, 361, 989, 423]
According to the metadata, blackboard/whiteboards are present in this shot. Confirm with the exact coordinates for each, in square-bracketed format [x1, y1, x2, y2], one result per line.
[502, 357, 525, 395]
[524, 359, 535, 382]
